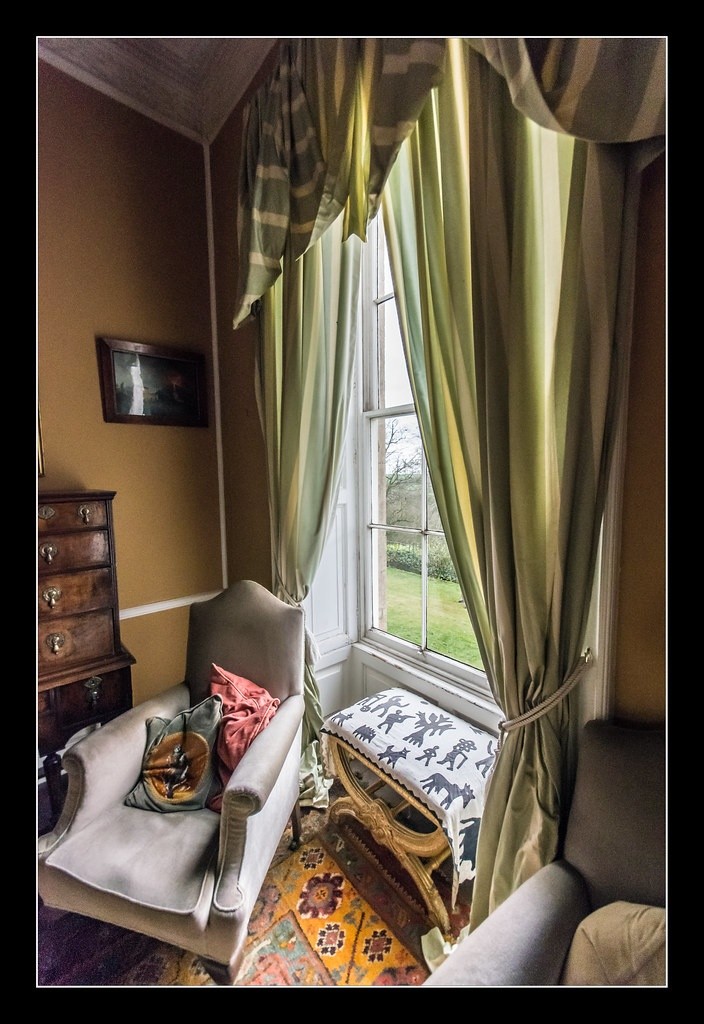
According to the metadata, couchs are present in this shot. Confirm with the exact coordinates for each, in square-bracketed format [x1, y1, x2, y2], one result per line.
[415, 718, 668, 988]
[35, 579, 307, 986]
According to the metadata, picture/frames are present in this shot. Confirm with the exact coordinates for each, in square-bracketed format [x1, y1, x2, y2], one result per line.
[94, 333, 210, 430]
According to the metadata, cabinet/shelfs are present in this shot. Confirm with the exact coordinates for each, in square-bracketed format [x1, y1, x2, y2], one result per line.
[37, 488, 136, 755]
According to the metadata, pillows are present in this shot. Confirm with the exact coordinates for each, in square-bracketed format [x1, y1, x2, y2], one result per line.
[124, 692, 222, 814]
[202, 658, 282, 814]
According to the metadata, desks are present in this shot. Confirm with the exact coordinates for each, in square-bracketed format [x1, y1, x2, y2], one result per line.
[321, 687, 502, 945]
